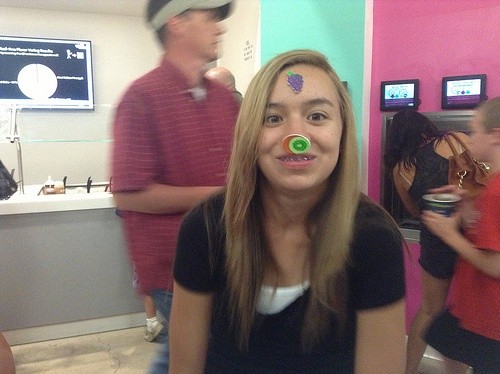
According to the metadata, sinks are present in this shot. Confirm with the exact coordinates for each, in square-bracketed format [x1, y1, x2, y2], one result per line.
[36, 183, 112, 196]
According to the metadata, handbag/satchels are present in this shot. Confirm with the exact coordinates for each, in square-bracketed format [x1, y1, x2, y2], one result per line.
[443, 133, 491, 192]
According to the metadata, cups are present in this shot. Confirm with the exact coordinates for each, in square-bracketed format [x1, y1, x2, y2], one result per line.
[422, 193, 462, 218]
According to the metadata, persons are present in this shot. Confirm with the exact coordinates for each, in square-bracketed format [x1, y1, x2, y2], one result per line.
[204, 67, 244, 111]
[168, 48, 403, 374]
[403, 94, 500, 374]
[385, 108, 477, 374]
[110, 0, 249, 374]
[144, 291, 166, 343]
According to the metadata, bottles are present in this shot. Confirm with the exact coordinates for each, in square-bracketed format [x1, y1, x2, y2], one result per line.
[54, 181, 64, 194]
[44, 185, 55, 195]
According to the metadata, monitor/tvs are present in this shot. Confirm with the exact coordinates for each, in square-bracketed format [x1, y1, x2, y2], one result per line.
[380, 79, 418, 111]
[0, 35, 93, 110]
[442, 74, 486, 109]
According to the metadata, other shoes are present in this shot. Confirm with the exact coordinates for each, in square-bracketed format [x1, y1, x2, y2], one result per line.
[144, 323, 168, 342]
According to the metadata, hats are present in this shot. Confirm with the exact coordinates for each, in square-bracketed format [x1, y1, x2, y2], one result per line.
[146, 0, 237, 32]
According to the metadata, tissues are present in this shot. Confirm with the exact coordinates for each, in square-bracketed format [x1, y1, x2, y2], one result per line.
[42, 176, 65, 194]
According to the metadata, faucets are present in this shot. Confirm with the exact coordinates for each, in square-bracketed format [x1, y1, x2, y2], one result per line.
[10, 104, 25, 194]
